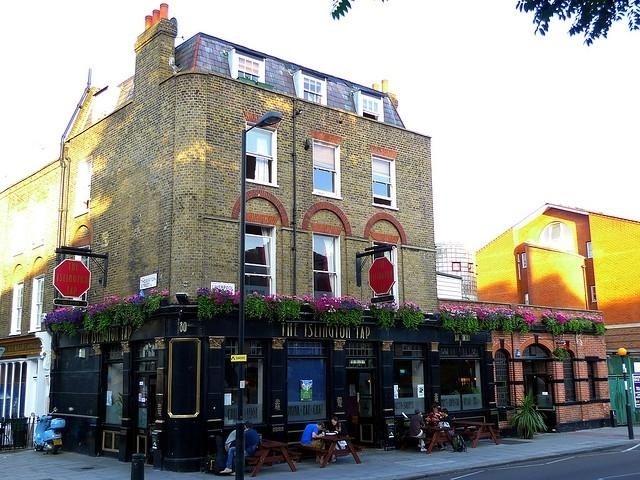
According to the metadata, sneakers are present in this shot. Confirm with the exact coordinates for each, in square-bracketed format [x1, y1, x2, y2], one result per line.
[418, 443, 428, 452]
[315, 458, 337, 468]
[220, 467, 236, 476]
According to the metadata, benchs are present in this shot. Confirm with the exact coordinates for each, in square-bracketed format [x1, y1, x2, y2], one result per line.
[464, 430, 479, 439]
[274, 447, 302, 462]
[445, 430, 461, 440]
[339, 441, 364, 451]
[305, 441, 328, 457]
[483, 428, 501, 436]
[413, 434, 432, 445]
[243, 452, 257, 467]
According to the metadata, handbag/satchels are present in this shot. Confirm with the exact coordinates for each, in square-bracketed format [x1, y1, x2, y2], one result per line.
[436, 418, 451, 429]
[335, 440, 348, 450]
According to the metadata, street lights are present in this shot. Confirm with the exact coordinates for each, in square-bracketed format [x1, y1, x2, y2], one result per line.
[237, 111, 284, 420]
[617, 348, 629, 404]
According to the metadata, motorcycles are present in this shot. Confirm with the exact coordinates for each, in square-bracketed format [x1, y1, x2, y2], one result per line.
[31, 407, 66, 454]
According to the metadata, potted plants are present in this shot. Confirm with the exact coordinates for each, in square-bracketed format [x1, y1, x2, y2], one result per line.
[508, 392, 548, 439]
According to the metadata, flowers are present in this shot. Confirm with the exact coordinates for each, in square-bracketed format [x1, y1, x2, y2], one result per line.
[198, 285, 424, 331]
[435, 301, 607, 338]
[42, 286, 170, 339]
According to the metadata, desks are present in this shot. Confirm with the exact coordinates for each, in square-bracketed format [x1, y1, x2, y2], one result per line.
[457, 418, 498, 448]
[319, 431, 362, 467]
[424, 423, 457, 454]
[251, 438, 297, 477]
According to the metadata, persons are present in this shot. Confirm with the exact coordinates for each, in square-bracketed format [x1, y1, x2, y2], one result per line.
[301, 422, 329, 465]
[426, 407, 444, 425]
[225, 428, 237, 452]
[439, 408, 456, 450]
[326, 417, 349, 462]
[219, 422, 259, 476]
[409, 410, 429, 451]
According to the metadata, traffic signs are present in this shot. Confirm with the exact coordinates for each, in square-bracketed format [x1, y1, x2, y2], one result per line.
[632, 372, 640, 408]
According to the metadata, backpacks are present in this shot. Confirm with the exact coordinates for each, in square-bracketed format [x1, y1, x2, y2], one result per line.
[452, 435, 465, 452]
